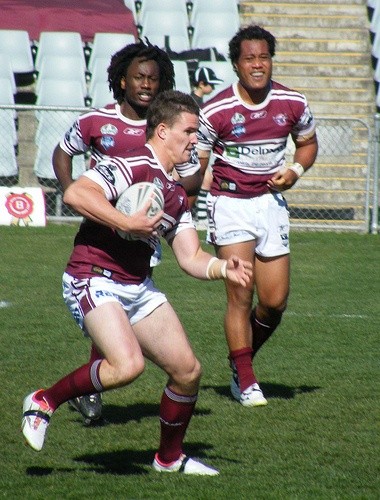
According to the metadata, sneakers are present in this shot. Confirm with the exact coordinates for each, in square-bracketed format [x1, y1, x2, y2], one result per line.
[229, 354, 267, 407]
[152, 452, 219, 475]
[21, 389, 54, 452]
[68, 392, 103, 425]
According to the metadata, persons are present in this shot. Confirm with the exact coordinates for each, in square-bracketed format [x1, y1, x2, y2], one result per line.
[190, 66, 224, 219]
[53, 43, 203, 427]
[20, 91, 254, 477]
[188, 25, 318, 407]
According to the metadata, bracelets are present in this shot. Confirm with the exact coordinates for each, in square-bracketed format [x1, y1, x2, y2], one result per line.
[289, 162, 304, 178]
[206, 255, 227, 279]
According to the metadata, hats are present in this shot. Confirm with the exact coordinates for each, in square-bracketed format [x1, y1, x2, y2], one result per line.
[192, 66, 224, 84]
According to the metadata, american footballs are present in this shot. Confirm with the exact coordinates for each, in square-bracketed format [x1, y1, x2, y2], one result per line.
[116, 181, 166, 242]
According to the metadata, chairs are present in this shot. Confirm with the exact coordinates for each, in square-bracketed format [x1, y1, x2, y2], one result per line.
[0, 0, 241, 216]
